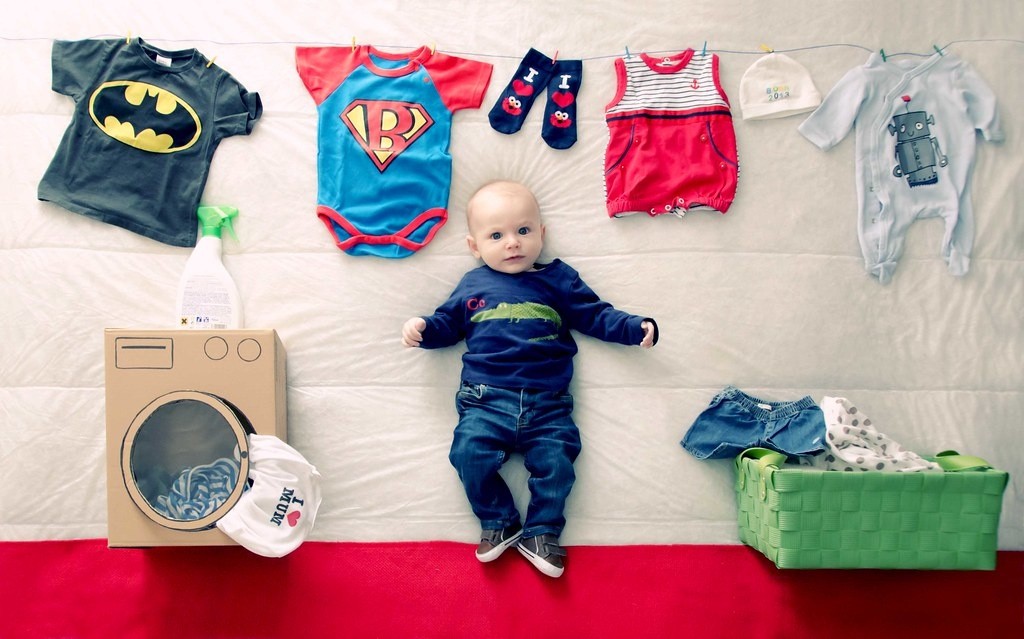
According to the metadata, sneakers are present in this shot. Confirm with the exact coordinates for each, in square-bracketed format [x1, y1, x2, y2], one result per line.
[517, 532, 565, 577]
[476, 523, 524, 562]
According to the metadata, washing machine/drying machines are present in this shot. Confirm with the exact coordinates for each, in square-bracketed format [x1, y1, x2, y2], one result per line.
[100, 326, 293, 553]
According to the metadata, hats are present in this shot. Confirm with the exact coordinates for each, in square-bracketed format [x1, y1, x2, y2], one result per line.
[739, 54, 819, 124]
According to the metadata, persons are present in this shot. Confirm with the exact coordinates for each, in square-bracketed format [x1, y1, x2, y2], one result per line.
[402, 179, 660, 578]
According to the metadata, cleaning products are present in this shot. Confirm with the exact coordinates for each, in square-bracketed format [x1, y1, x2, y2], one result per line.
[169, 203, 247, 332]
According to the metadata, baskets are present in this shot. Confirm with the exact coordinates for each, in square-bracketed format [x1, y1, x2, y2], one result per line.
[732, 449, 1010, 573]
